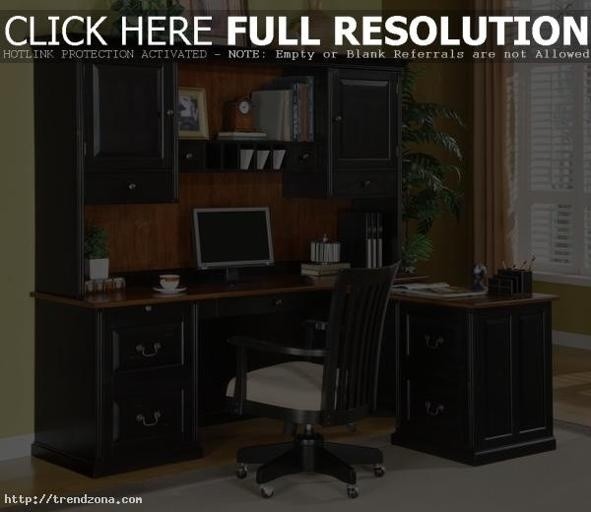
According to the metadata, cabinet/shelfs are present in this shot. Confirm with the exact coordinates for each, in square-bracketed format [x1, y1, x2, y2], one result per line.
[331, 69, 405, 203]
[75, 60, 178, 204]
[403, 304, 467, 462]
[99, 313, 190, 475]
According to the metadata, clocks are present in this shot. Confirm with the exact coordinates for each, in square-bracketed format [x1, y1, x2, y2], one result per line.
[231, 96, 256, 132]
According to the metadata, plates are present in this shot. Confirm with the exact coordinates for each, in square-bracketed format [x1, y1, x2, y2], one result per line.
[153, 286, 187, 294]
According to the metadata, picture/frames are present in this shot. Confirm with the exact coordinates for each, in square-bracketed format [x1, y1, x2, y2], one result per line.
[180, 86, 210, 141]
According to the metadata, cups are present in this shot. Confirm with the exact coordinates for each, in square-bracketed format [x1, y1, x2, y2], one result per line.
[159, 275, 181, 289]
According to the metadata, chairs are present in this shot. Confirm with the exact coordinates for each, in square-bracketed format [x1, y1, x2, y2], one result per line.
[222, 258, 406, 499]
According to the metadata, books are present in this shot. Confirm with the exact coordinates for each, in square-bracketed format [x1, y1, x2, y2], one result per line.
[302, 261, 353, 271]
[299, 268, 340, 277]
[253, 73, 316, 142]
[393, 281, 487, 298]
[339, 211, 372, 269]
[369, 212, 379, 267]
[376, 212, 385, 267]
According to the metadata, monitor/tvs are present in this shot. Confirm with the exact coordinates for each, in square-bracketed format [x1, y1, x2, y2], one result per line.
[194, 206, 275, 283]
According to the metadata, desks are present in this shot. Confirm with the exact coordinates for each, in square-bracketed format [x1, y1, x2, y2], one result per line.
[32, 268, 568, 316]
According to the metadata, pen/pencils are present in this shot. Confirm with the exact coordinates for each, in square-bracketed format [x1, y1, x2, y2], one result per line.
[501, 255, 536, 272]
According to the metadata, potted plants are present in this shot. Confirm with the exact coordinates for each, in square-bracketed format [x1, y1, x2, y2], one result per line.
[66, 224, 112, 294]
